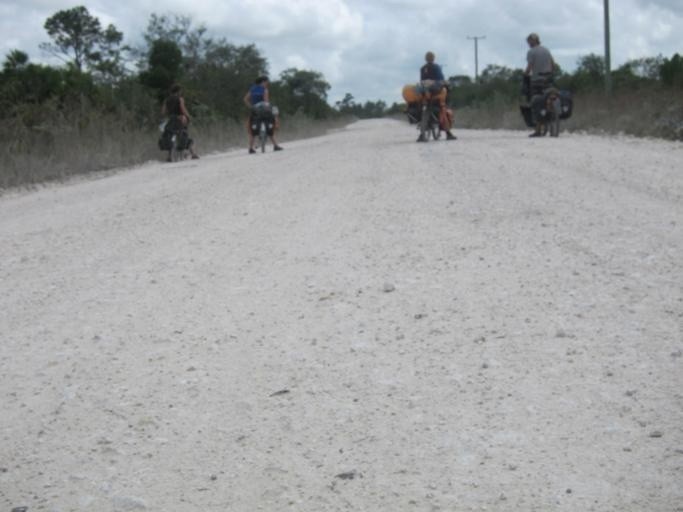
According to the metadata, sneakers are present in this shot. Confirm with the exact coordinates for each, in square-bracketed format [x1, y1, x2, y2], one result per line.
[192, 155, 199, 158]
[529, 132, 540, 137]
[274, 146, 283, 150]
[250, 149, 256, 153]
[446, 135, 456, 139]
[416, 135, 424, 141]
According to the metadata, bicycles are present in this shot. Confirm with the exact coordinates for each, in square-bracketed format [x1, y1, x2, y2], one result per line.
[169, 131, 189, 162]
[538, 95, 562, 137]
[420, 102, 442, 144]
[259, 121, 267, 153]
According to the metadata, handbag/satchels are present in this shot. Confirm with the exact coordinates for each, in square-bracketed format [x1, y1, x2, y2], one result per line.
[249, 109, 275, 137]
[533, 88, 573, 122]
[158, 116, 192, 150]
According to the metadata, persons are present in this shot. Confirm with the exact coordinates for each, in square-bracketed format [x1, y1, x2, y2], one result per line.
[416, 52, 457, 142]
[161, 83, 199, 161]
[523, 33, 556, 137]
[243, 76, 283, 154]
